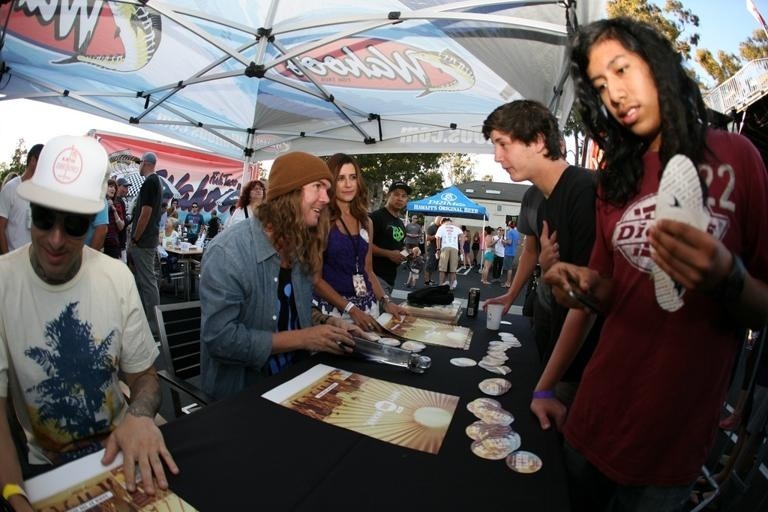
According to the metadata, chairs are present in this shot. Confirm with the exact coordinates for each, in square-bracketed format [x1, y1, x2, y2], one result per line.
[154, 300, 217, 419]
[155, 227, 204, 300]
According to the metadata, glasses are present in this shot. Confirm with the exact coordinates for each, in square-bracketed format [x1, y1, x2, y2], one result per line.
[192, 205, 198, 208]
[29, 201, 98, 237]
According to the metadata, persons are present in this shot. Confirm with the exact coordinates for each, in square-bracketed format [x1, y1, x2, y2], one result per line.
[482, 99, 603, 447]
[366, 182, 413, 298]
[84, 178, 266, 285]
[199, 152, 371, 402]
[0, 144, 44, 254]
[0, 135, 180, 512]
[542, 15, 768, 512]
[692, 330, 768, 512]
[312, 153, 412, 345]
[126, 151, 162, 346]
[404, 207, 563, 350]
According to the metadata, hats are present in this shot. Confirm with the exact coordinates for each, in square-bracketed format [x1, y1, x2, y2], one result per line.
[116, 177, 133, 186]
[266, 151, 335, 203]
[387, 181, 413, 195]
[135, 152, 157, 164]
[15, 134, 112, 215]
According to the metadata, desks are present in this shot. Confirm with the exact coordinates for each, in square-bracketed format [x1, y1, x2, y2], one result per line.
[0, 301, 579, 512]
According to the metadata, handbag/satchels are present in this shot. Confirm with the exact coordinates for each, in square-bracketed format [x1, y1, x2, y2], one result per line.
[407, 285, 454, 307]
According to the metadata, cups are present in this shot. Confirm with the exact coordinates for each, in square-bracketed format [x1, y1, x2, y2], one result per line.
[167, 240, 172, 245]
[487, 304, 504, 330]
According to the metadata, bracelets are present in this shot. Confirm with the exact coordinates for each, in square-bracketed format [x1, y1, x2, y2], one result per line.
[533, 390, 557, 399]
[3, 483, 30, 499]
[343, 300, 354, 314]
[380, 294, 392, 309]
[712, 253, 746, 302]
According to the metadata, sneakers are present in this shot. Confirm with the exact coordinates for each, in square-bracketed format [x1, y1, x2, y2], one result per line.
[402, 280, 458, 291]
[481, 279, 510, 288]
